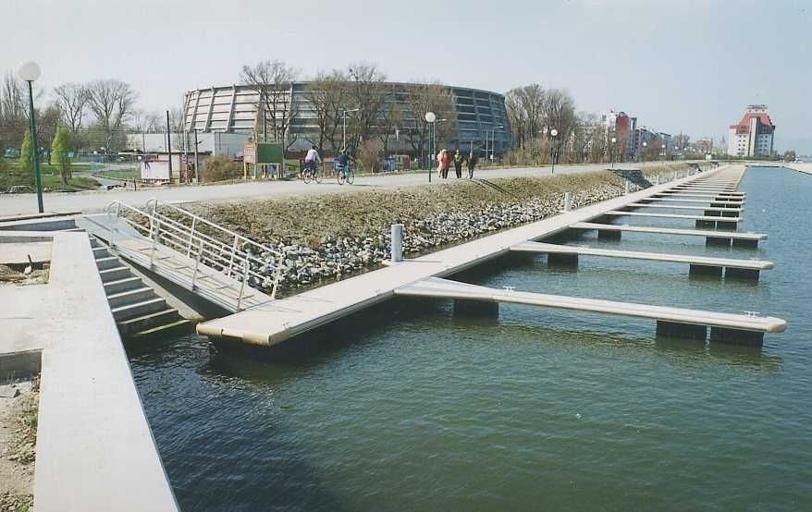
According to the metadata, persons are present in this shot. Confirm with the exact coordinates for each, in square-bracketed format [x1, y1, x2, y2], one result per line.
[304, 145, 322, 180]
[436, 148, 449, 179]
[464, 152, 475, 180]
[337, 149, 354, 184]
[452, 149, 463, 178]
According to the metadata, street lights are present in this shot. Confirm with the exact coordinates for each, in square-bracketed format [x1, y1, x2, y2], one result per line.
[342, 108, 360, 155]
[19, 58, 46, 214]
[492, 125, 503, 164]
[610, 137, 617, 168]
[434, 118, 447, 169]
[426, 111, 435, 182]
[641, 141, 647, 166]
[661, 144, 696, 163]
[549, 129, 558, 173]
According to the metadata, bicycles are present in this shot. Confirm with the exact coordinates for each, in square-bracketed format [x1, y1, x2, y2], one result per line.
[335, 164, 354, 185]
[302, 163, 323, 184]
